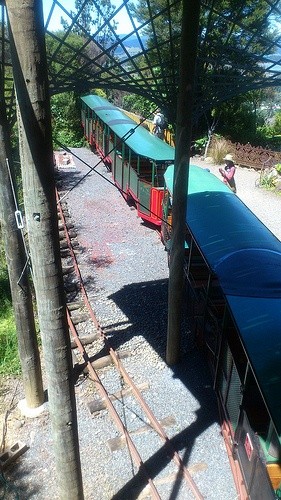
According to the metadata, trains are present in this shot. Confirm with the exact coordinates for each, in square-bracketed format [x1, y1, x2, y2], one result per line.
[82, 94, 281, 500]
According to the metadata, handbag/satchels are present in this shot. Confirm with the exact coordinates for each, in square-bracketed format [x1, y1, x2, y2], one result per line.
[157, 128, 165, 139]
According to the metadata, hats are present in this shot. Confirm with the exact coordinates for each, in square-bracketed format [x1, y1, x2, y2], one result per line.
[222, 154, 235, 163]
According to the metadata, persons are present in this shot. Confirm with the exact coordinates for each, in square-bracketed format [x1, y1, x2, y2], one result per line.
[219, 154, 236, 193]
[138, 116, 149, 132]
[152, 107, 167, 139]
